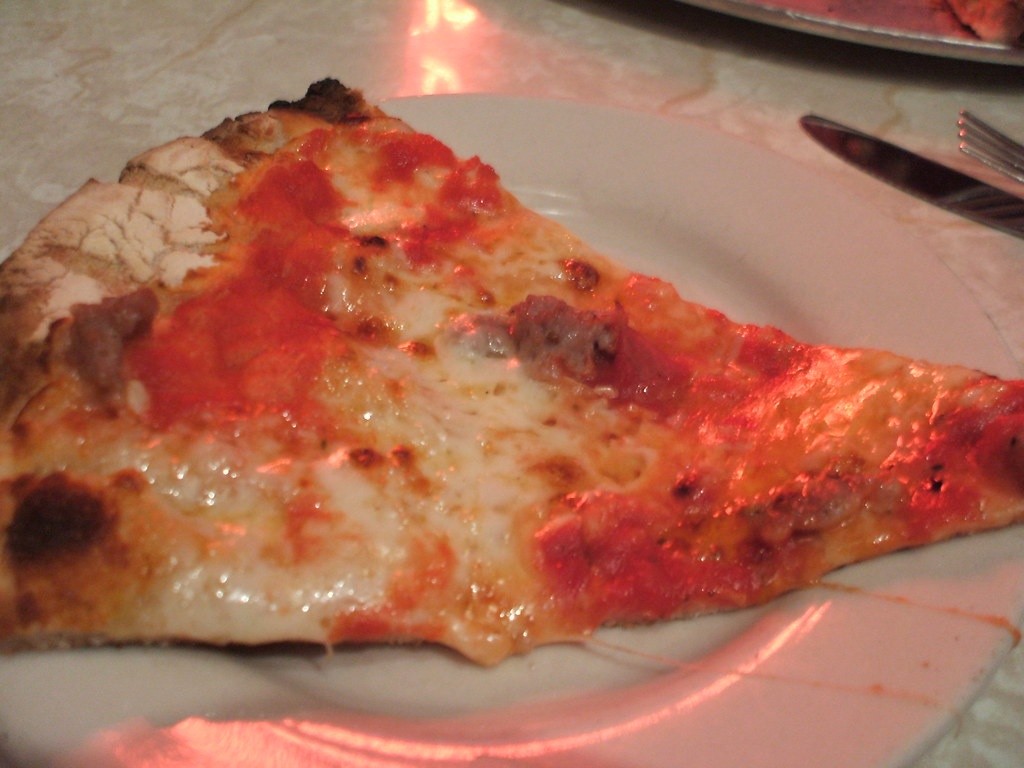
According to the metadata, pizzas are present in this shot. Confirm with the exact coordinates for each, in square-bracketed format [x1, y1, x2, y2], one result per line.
[0, 76, 1023, 663]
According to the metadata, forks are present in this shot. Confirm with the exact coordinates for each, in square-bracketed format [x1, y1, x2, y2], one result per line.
[956, 109, 1024, 183]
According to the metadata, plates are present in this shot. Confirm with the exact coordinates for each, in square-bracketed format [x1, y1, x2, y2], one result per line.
[684, 0, 1024, 66]
[0, 94, 1024, 768]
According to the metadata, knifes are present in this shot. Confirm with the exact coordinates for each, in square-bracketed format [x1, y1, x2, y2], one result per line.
[799, 115, 1024, 237]
